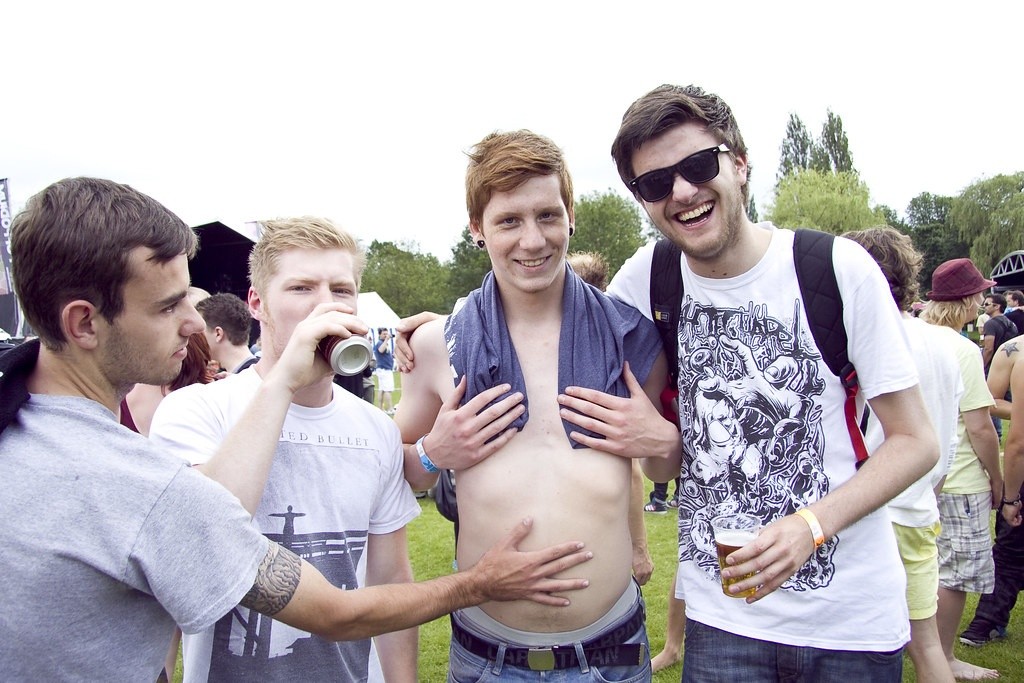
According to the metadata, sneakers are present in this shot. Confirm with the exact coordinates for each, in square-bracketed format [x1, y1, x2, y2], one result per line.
[959, 618, 1006, 647]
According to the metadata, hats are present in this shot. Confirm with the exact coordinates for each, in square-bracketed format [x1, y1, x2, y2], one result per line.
[925, 258, 997, 301]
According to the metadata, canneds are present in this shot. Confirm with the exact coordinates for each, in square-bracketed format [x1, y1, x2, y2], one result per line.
[318, 330, 372, 376]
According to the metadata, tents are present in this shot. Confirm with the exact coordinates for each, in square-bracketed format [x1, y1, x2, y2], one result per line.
[355, 292, 402, 327]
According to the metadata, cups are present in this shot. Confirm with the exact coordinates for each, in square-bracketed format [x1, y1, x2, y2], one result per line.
[710, 513, 762, 599]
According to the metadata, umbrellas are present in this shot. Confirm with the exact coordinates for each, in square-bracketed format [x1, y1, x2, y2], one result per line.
[184, 222, 260, 349]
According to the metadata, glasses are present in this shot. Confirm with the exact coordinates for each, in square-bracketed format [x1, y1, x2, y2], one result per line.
[628, 143, 731, 203]
[984, 303, 997, 306]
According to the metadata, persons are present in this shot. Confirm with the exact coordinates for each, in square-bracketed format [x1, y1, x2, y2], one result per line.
[0, 177, 593, 683]
[123, 86, 1024, 683]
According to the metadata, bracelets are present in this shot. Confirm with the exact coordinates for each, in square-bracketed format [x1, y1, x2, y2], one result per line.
[416, 432, 438, 474]
[1002, 493, 1021, 504]
[795, 508, 826, 551]
